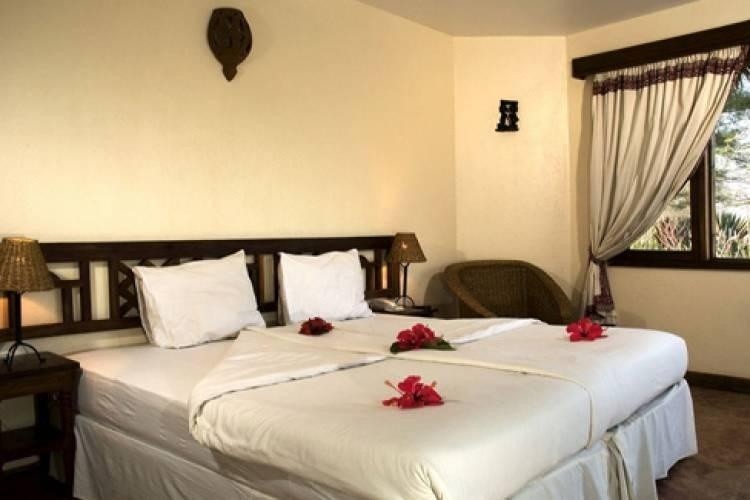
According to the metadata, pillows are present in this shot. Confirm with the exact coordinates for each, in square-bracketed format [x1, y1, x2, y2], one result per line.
[130, 248, 374, 349]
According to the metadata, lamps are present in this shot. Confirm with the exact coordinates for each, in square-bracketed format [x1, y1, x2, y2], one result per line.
[0, 236, 56, 371]
[386, 232, 427, 306]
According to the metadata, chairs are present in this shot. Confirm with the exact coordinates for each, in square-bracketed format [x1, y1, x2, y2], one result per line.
[443, 260, 577, 324]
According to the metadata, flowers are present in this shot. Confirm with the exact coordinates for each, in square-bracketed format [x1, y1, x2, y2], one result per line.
[22, 235, 689, 499]
[383, 374, 444, 409]
[390, 324, 456, 353]
[566, 319, 608, 342]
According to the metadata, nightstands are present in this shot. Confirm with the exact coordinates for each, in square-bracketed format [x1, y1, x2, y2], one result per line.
[0, 351, 80, 499]
